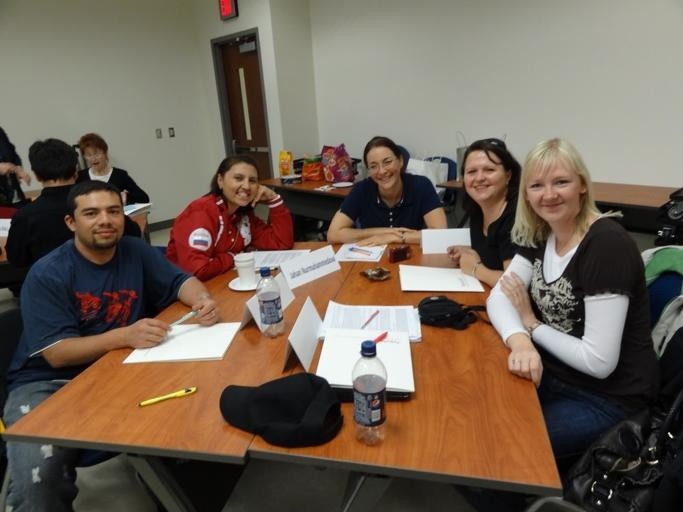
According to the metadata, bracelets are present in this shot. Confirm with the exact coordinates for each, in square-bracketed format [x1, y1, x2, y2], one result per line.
[528, 321, 543, 336]
[8, 165, 18, 172]
[200, 296, 209, 300]
[272, 191, 276, 198]
[401, 231, 405, 243]
[472, 261, 482, 277]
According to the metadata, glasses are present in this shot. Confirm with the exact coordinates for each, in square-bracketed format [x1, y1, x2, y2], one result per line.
[488, 138, 506, 152]
[367, 159, 394, 174]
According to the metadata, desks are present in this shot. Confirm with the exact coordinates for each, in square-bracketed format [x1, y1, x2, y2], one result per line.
[248, 240, 565, 512]
[436, 171, 680, 237]
[119, 199, 151, 239]
[256, 175, 357, 222]
[0, 223, 354, 512]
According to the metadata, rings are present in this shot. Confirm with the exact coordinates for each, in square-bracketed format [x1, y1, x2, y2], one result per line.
[211, 311, 216, 317]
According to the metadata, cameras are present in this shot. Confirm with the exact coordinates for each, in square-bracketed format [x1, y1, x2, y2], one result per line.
[390, 245, 411, 263]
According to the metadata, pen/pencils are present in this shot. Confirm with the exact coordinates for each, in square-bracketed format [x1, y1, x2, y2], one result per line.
[135, 387, 197, 407]
[349, 247, 376, 256]
[361, 311, 380, 329]
[373, 331, 388, 343]
[169, 310, 200, 328]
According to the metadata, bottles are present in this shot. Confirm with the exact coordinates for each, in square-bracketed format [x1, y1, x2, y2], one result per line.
[255, 268, 285, 337]
[351, 339, 388, 447]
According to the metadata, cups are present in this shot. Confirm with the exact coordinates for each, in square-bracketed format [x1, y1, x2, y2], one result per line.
[234, 252, 256, 287]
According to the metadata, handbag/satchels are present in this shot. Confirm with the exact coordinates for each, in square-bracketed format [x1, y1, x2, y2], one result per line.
[418, 295, 476, 330]
[563, 403, 682, 512]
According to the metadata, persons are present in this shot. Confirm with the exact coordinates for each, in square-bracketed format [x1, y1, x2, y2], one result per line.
[327, 135, 448, 247]
[164, 155, 294, 283]
[485, 138, 661, 470]
[446, 139, 522, 288]
[3, 179, 221, 512]
[75, 134, 149, 205]
[4, 139, 142, 298]
[0, 127, 30, 207]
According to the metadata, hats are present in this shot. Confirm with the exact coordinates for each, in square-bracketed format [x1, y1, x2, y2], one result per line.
[219, 372, 344, 449]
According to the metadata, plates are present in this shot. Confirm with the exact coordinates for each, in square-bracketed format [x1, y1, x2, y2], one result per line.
[332, 180, 353, 189]
[226, 277, 258, 292]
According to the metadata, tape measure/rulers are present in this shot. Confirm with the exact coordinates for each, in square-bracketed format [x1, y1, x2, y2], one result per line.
[291, 257, 335, 278]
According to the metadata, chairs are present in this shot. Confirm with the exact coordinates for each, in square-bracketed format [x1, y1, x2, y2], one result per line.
[521, 244, 681, 512]
[0, 306, 122, 512]
[421, 156, 459, 215]
